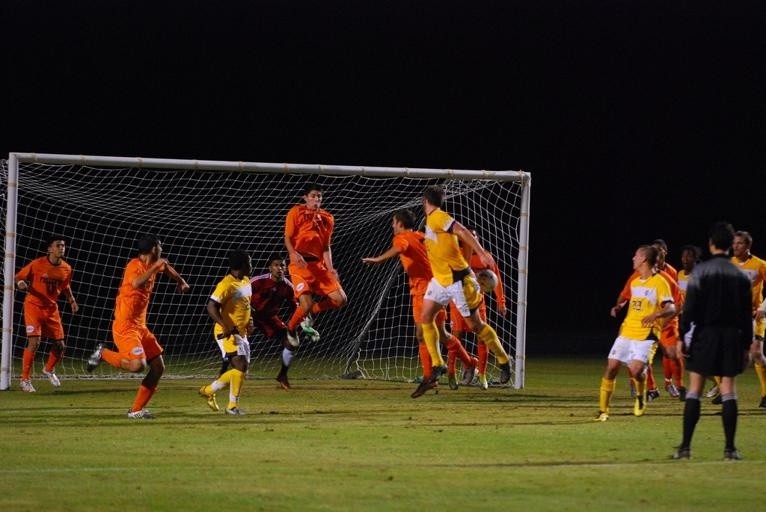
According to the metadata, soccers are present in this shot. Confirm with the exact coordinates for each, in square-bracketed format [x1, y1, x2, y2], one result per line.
[477, 268, 499, 292]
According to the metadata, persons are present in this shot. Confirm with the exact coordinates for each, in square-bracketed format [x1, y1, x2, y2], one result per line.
[250, 255, 304, 390]
[593, 230, 766, 422]
[15, 238, 80, 393]
[668, 221, 753, 460]
[447, 229, 493, 391]
[87, 235, 190, 419]
[362, 211, 479, 398]
[199, 252, 254, 416]
[281, 186, 347, 348]
[419, 188, 513, 382]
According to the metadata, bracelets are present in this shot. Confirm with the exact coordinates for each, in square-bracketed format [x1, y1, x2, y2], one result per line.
[18, 280, 24, 286]
[69, 295, 75, 302]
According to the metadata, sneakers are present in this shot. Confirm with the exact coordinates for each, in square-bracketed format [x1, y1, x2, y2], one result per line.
[18, 320, 762, 462]
